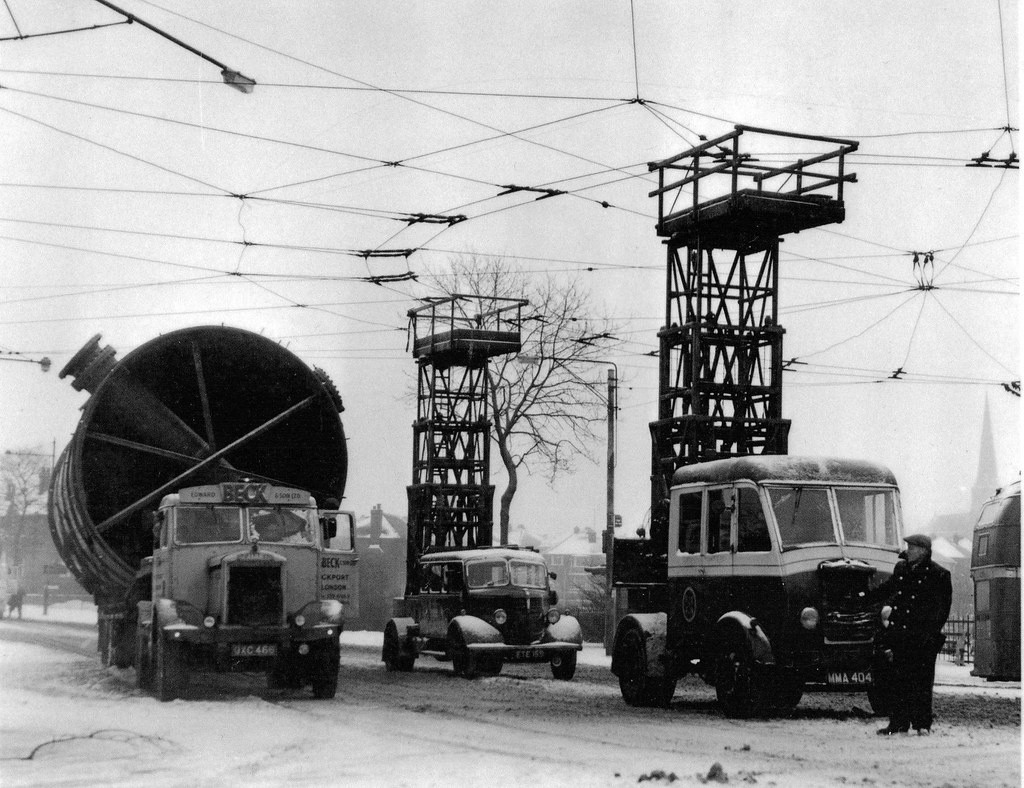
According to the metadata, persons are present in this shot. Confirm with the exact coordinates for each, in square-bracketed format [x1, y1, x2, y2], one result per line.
[866, 534, 953, 735]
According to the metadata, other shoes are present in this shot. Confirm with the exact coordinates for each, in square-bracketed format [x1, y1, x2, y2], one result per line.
[877, 725, 930, 740]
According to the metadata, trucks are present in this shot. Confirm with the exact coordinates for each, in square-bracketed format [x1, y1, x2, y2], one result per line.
[96, 480, 358, 701]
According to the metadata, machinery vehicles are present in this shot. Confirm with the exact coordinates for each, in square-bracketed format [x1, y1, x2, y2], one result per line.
[384, 549, 583, 679]
[610, 454, 912, 716]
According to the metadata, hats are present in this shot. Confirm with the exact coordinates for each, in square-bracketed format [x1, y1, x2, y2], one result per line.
[903, 534, 935, 549]
